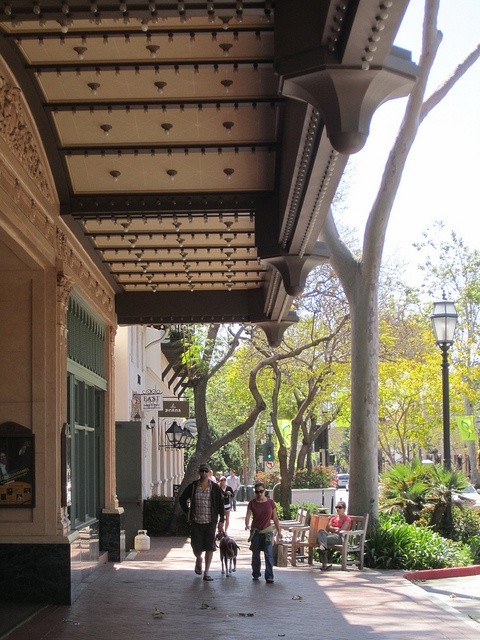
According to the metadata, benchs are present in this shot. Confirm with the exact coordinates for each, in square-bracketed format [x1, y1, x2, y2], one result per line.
[299, 514, 369, 571]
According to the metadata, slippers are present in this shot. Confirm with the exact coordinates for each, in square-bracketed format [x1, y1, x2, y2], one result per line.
[204, 571, 213, 580]
[195, 564, 202, 574]
[320, 565, 332, 570]
[317, 549, 327, 554]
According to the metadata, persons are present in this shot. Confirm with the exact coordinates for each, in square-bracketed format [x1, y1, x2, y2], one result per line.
[316, 502, 351, 570]
[0, 440, 31, 485]
[208, 470, 216, 483]
[179, 464, 224, 580]
[245, 483, 282, 582]
[218, 476, 234, 532]
[226, 469, 240, 511]
[215, 473, 221, 481]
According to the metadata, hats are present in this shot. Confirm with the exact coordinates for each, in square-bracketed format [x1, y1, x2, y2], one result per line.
[199, 463, 211, 472]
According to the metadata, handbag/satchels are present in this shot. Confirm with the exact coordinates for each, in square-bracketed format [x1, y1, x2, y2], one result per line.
[273, 544, 288, 567]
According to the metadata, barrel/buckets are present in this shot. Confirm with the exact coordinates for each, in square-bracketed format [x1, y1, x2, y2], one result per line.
[134, 529, 151, 550]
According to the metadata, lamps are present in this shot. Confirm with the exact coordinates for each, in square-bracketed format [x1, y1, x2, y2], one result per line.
[235, 13, 243, 26]
[146, 276, 152, 283]
[213, 64, 220, 74]
[365, 54, 372, 63]
[211, 31, 217, 44]
[215, 105, 221, 113]
[162, 105, 166, 114]
[188, 285, 196, 292]
[171, 426, 189, 449]
[123, 12, 130, 24]
[206, 1, 215, 11]
[198, 104, 202, 111]
[91, 1, 97, 11]
[99, 124, 113, 138]
[10, 17, 17, 28]
[183, 265, 190, 272]
[326, 44, 335, 54]
[145, 33, 153, 45]
[128, 240, 137, 248]
[188, 435, 195, 449]
[139, 18, 148, 31]
[224, 252, 233, 260]
[331, 34, 338, 43]
[372, 34, 380, 44]
[223, 121, 235, 136]
[334, 25, 339, 32]
[254, 31, 261, 44]
[220, 80, 233, 94]
[154, 66, 162, 77]
[278, 149, 340, 322]
[234, 0, 244, 11]
[148, 2, 157, 11]
[145, 419, 156, 429]
[38, 38, 44, 47]
[150, 12, 158, 22]
[381, 0, 393, 9]
[194, 64, 199, 76]
[140, 265, 148, 273]
[80, 34, 87, 45]
[376, 22, 383, 31]
[220, 17, 232, 31]
[121, 223, 131, 232]
[95, 69, 100, 79]
[146, 45, 160, 58]
[186, 276, 193, 283]
[176, 429, 193, 449]
[173, 223, 182, 232]
[93, 13, 101, 25]
[225, 264, 233, 272]
[124, 34, 130, 46]
[179, 12, 188, 25]
[189, 32, 195, 45]
[176, 239, 185, 247]
[379, 11, 388, 20]
[56, 69, 62, 78]
[265, 109, 320, 322]
[61, 0, 69, 14]
[33, 0, 41, 14]
[88, 82, 101, 95]
[232, 32, 239, 44]
[155, 81, 167, 94]
[368, 43, 377, 54]
[77, 70, 82, 78]
[337, 14, 342, 23]
[135, 253, 143, 261]
[232, 63, 240, 74]
[224, 168, 233, 181]
[160, 123, 173, 136]
[264, 14, 270, 21]
[167, 31, 174, 45]
[338, 3, 345, 11]
[120, 0, 127, 12]
[160, 420, 183, 450]
[178, 1, 186, 13]
[67, 16, 75, 26]
[73, 47, 88, 62]
[225, 238, 233, 247]
[251, 103, 257, 112]
[173, 64, 180, 75]
[102, 33, 108, 47]
[252, 63, 259, 74]
[224, 222, 233, 231]
[180, 253, 188, 261]
[179, 103, 185, 114]
[219, 43, 233, 56]
[39, 16, 47, 27]
[58, 19, 70, 34]
[208, 13, 216, 24]
[263, 2, 272, 11]
[234, 103, 238, 112]
[166, 169, 177, 180]
[134, 66, 140, 76]
[151, 286, 156, 292]
[3, 0, 12, 16]
[60, 37, 65, 46]
[109, 170, 121, 182]
[361, 62, 370, 71]
[226, 275, 233, 282]
[226, 285, 232, 291]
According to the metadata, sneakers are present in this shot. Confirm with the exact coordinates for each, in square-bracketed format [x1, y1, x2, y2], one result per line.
[266, 575, 274, 583]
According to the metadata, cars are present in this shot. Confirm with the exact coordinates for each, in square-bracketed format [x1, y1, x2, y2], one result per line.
[451, 480, 480, 515]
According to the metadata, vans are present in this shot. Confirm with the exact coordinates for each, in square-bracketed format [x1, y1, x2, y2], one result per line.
[335, 473, 350, 489]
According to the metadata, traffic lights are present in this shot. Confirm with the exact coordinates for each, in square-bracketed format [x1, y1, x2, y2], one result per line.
[266, 445, 272, 459]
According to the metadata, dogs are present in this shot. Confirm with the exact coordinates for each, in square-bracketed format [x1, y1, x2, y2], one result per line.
[219, 536, 240, 578]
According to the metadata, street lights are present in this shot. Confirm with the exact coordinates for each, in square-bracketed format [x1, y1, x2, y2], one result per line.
[429, 289, 459, 472]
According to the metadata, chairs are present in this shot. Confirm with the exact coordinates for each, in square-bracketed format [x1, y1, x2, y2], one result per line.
[269, 509, 303, 551]
[273, 510, 309, 563]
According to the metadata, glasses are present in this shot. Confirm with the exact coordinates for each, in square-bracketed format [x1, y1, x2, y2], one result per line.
[254, 490, 265, 494]
[200, 470, 210, 473]
[335, 506, 342, 509]
